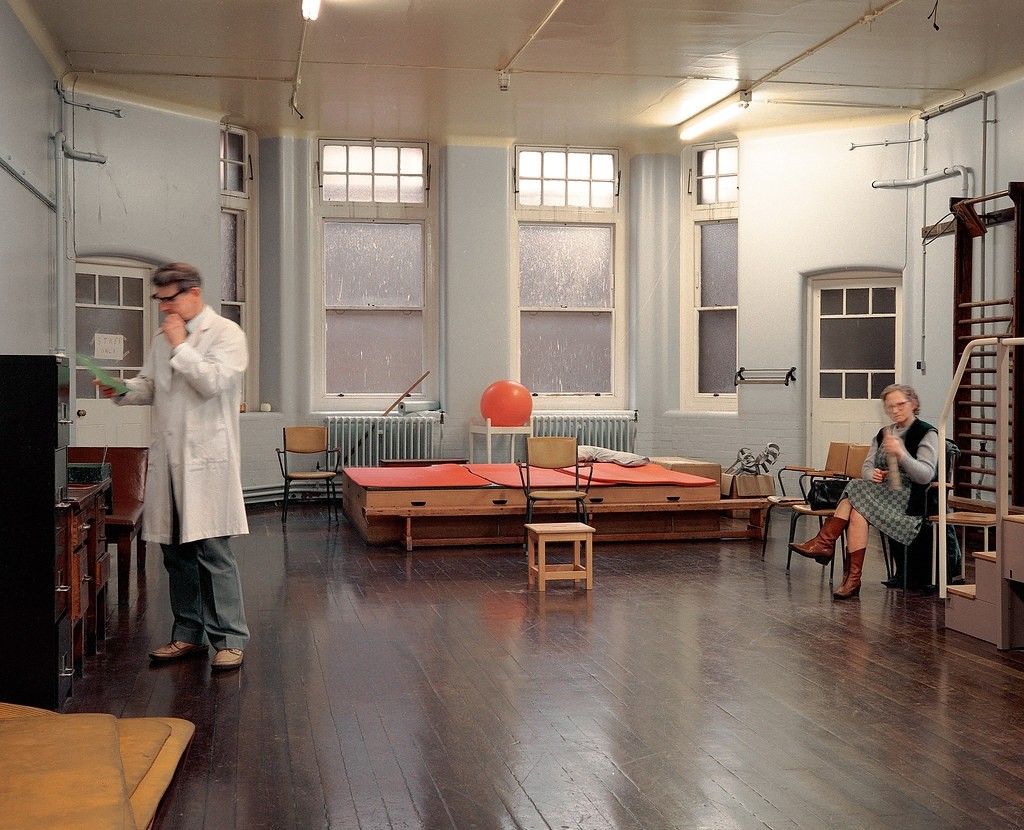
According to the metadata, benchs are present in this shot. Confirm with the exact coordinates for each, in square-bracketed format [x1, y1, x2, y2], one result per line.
[361, 498, 806, 550]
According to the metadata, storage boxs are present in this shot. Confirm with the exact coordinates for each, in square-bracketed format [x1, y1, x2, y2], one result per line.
[720, 467, 777, 518]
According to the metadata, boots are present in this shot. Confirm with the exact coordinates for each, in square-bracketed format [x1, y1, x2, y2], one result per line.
[832, 545, 867, 599]
[788, 516, 849, 565]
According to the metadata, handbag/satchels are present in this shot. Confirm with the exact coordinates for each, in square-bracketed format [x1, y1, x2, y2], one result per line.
[810, 479, 852, 511]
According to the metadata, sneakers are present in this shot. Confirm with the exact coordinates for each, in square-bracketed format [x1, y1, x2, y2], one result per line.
[211, 647, 243, 670]
[148, 640, 209, 660]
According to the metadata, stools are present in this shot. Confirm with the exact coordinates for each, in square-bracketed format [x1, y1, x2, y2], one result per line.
[927, 509, 996, 587]
[525, 523, 595, 592]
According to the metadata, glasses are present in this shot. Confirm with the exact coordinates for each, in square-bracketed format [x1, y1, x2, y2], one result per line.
[151, 288, 186, 305]
[886, 399, 911, 414]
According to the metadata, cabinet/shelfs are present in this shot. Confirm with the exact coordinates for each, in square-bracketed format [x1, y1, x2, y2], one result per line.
[0, 354, 72, 706]
[68, 479, 111, 655]
[340, 464, 721, 538]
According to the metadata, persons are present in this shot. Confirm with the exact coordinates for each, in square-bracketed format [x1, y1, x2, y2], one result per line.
[93, 262, 251, 670]
[787, 384, 939, 599]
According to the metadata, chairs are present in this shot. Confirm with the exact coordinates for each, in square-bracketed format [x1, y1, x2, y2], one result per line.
[761, 442, 850, 563]
[67, 446, 149, 600]
[276, 427, 341, 524]
[787, 444, 890, 588]
[515, 437, 593, 555]
[892, 440, 964, 596]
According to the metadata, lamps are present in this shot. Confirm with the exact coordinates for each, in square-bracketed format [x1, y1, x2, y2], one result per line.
[676, 90, 753, 141]
[300, 1, 320, 21]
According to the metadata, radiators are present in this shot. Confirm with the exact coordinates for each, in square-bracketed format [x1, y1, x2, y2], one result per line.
[532, 415, 638, 456]
[324, 418, 433, 472]
